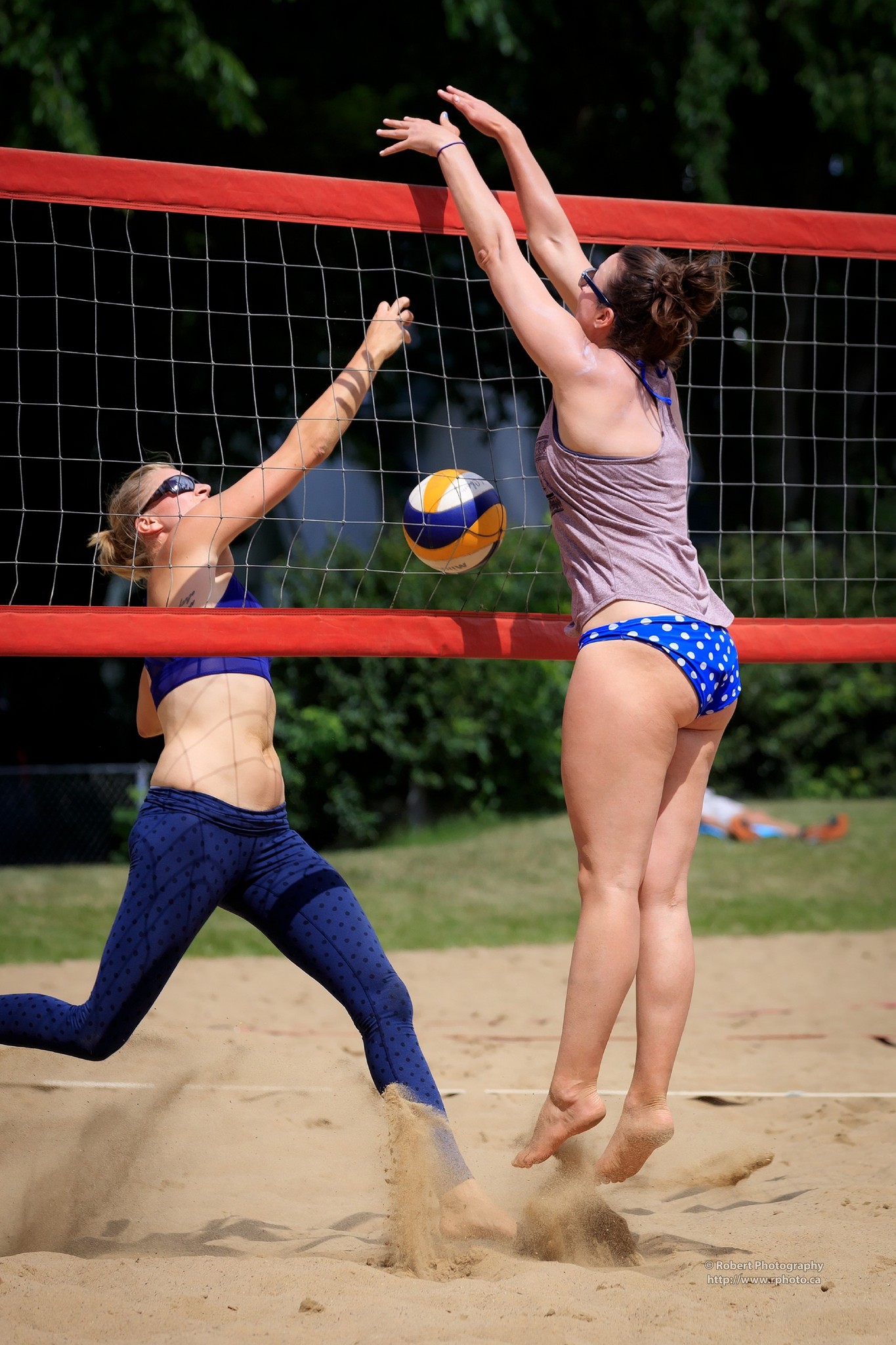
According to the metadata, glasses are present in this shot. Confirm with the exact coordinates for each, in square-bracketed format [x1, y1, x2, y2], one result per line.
[577, 268, 612, 307]
[141, 475, 199, 513]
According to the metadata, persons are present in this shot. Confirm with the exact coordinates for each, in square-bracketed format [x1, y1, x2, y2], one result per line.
[376, 86, 744, 1187]
[0, 297, 472, 1182]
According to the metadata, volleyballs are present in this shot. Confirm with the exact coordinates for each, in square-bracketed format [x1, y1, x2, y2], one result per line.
[402, 468, 507, 574]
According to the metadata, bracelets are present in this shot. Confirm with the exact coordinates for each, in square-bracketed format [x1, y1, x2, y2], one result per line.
[435, 140, 466, 163]
